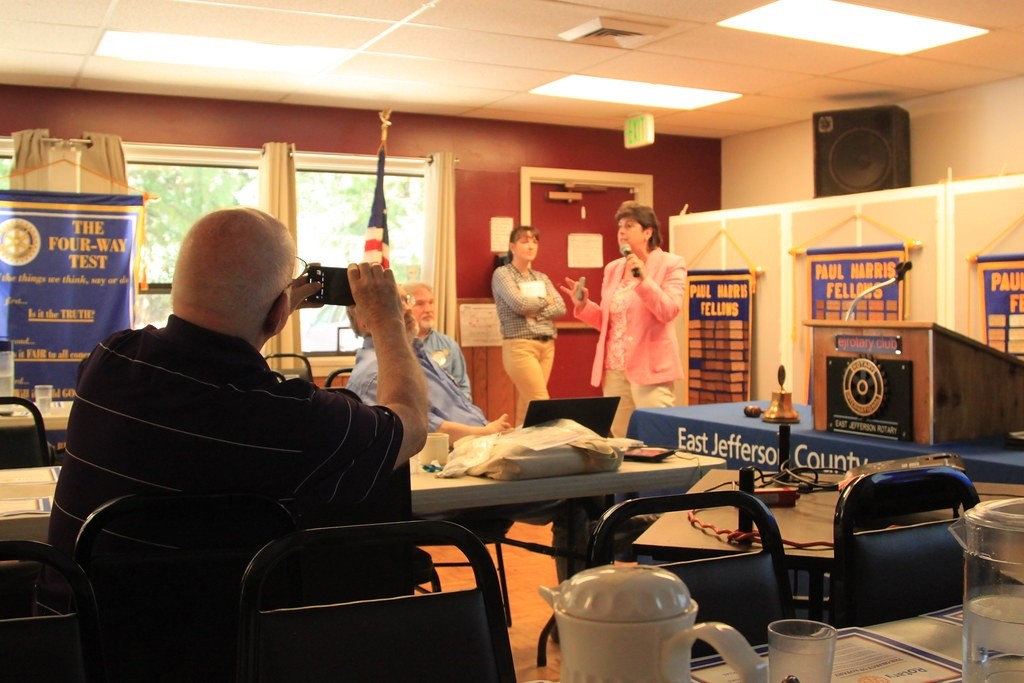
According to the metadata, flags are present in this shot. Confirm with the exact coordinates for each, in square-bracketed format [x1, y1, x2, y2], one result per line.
[364, 163, 389, 269]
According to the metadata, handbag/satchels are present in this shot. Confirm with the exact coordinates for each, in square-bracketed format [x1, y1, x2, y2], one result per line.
[454, 419, 624, 481]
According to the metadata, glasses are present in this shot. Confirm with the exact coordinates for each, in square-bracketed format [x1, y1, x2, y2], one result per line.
[269, 256, 310, 315]
[403, 292, 417, 308]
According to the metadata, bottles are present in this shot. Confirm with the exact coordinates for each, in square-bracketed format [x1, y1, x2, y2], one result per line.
[0, 340, 14, 416]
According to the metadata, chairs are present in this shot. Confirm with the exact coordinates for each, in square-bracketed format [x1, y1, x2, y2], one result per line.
[0, 353, 799, 683]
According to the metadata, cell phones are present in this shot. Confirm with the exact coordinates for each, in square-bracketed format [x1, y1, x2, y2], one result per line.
[306, 266, 355, 306]
[624, 448, 675, 462]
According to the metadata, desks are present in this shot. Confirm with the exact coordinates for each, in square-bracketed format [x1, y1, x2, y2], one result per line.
[691, 603, 964, 683]
[1, 401, 75, 430]
[629, 466, 1024, 623]
[1, 450, 727, 670]
[613, 397, 1024, 507]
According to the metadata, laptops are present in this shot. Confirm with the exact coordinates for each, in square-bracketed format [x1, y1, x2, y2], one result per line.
[522, 396, 620, 438]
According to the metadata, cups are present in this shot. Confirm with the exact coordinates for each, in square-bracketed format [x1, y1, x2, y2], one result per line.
[34, 385, 52, 416]
[419, 433, 449, 471]
[767, 619, 837, 683]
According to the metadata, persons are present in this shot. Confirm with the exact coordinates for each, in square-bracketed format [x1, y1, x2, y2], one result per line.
[491, 227, 566, 427]
[32, 209, 430, 617]
[560, 199, 689, 438]
[347, 284, 513, 450]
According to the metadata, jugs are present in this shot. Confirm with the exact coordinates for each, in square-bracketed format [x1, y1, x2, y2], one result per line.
[538, 557, 767, 683]
[949, 498, 1024, 683]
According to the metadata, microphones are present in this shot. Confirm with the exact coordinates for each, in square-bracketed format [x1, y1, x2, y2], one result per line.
[621, 243, 640, 277]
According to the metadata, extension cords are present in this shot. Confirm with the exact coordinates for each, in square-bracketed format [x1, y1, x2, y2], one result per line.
[755, 487, 800, 508]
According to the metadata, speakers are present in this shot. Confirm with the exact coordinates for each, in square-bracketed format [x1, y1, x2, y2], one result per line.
[812, 106, 911, 198]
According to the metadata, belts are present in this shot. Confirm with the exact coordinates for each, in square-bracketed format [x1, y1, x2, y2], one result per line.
[532, 336, 553, 343]
